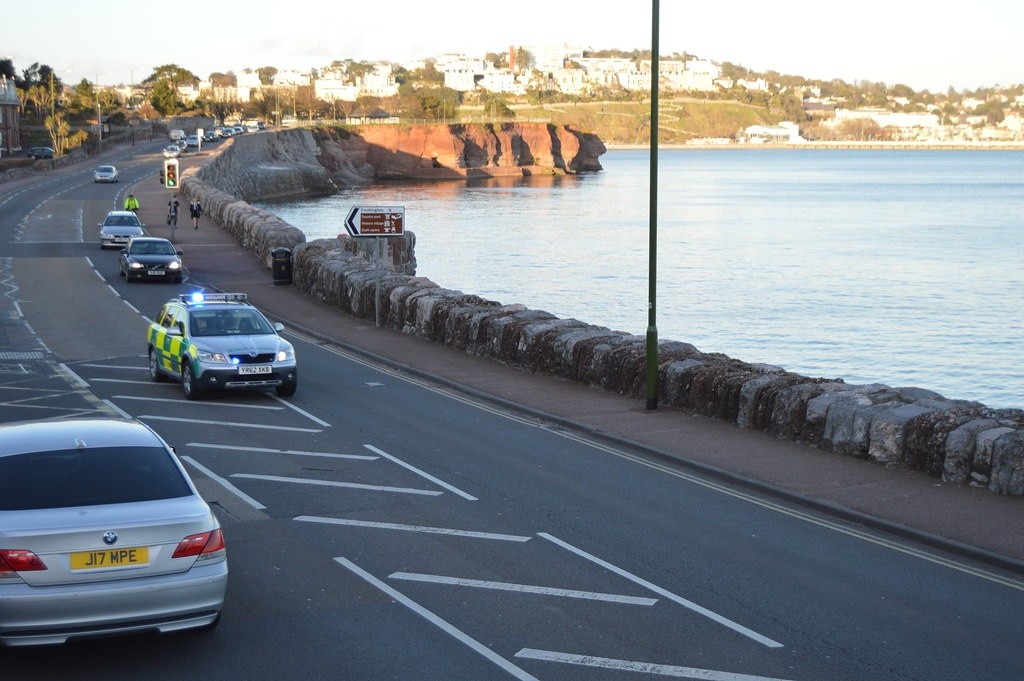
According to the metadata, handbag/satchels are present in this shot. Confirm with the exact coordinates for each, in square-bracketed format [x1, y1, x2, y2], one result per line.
[167, 215, 172, 225]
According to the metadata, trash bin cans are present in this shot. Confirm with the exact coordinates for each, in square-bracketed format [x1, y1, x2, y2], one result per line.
[272, 247, 292, 286]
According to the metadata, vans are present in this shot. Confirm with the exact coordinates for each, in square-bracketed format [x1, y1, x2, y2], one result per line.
[170, 130, 187, 141]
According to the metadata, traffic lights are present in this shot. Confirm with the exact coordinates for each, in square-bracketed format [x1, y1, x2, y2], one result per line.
[164, 160, 179, 188]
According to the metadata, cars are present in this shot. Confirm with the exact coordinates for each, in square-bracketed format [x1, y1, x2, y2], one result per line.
[146, 293, 298, 400]
[173, 140, 188, 152]
[202, 132, 219, 142]
[187, 135, 203, 147]
[0, 416, 230, 647]
[118, 236, 184, 284]
[27, 147, 54, 160]
[97, 211, 147, 249]
[163, 146, 180, 158]
[93, 165, 119, 183]
[214, 121, 265, 138]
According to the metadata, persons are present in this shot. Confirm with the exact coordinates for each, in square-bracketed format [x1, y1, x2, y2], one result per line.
[189, 195, 202, 231]
[124, 192, 139, 212]
[167, 195, 180, 229]
